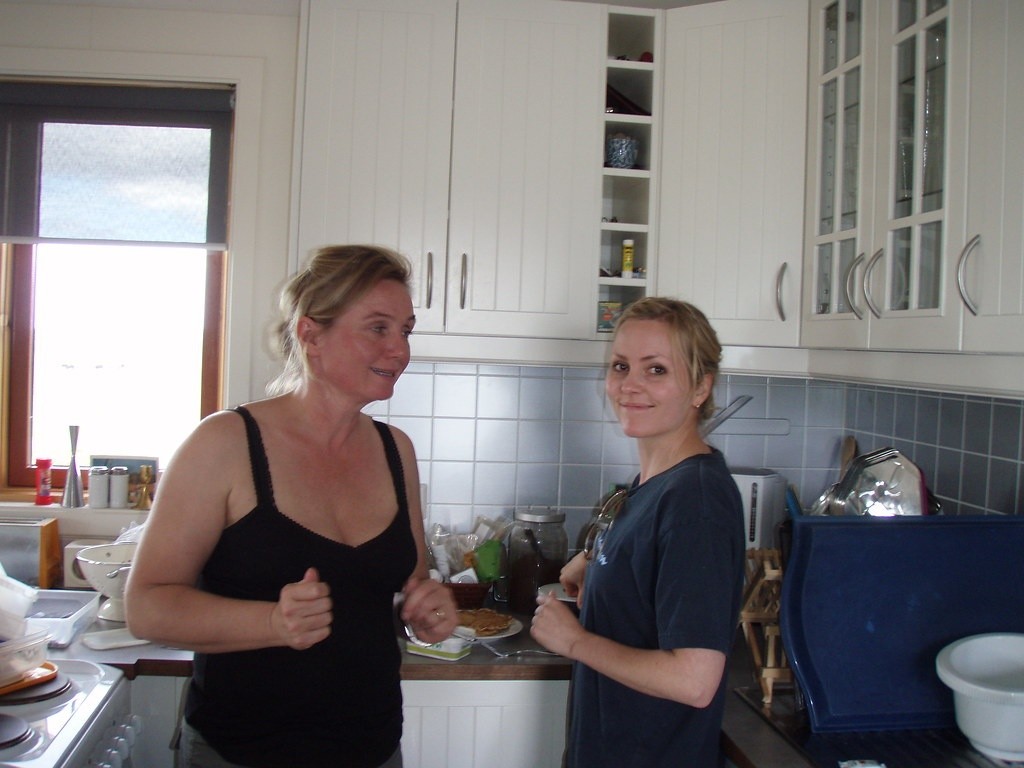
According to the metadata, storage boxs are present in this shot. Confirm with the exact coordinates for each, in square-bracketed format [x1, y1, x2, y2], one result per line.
[597, 301, 623, 332]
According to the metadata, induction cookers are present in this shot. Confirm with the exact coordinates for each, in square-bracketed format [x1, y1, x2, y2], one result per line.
[0, 658, 143, 768]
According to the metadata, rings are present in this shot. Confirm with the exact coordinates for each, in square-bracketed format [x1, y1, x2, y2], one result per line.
[431, 607, 446, 622]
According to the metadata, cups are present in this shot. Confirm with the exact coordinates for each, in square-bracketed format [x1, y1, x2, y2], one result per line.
[607, 138, 640, 168]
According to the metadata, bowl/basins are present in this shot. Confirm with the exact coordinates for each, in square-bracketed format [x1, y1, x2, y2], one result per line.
[936, 632, 1024, 761]
[75, 541, 138, 623]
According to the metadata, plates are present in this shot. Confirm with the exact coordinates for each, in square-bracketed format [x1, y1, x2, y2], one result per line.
[538, 583, 579, 603]
[450, 609, 524, 644]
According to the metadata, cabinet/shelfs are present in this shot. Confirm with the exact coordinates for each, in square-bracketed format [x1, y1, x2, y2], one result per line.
[654, 0, 809, 381]
[804, 0, 1024, 401]
[284, 0, 664, 370]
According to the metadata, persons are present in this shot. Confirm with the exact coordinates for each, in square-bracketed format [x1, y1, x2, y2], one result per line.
[123, 244, 460, 768]
[530, 297, 746, 768]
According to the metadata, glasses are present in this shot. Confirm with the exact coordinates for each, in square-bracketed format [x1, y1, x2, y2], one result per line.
[583, 489, 628, 560]
